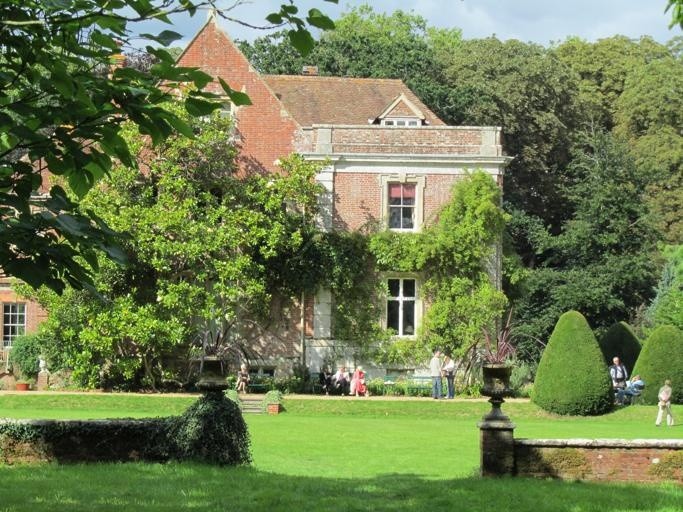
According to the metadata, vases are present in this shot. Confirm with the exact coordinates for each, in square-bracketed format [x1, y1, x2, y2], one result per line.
[15, 384, 30, 390]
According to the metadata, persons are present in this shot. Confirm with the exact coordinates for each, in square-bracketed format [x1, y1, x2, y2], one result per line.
[349, 367, 369, 396]
[429, 348, 447, 399]
[614, 375, 645, 406]
[318, 366, 334, 395]
[331, 364, 349, 395]
[608, 356, 629, 391]
[442, 352, 457, 398]
[654, 379, 675, 428]
[236, 364, 251, 395]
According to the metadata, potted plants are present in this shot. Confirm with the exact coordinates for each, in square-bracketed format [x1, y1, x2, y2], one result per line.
[462, 306, 547, 397]
[187, 318, 266, 382]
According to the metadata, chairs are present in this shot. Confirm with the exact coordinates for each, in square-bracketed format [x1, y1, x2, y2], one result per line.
[628, 384, 644, 405]
